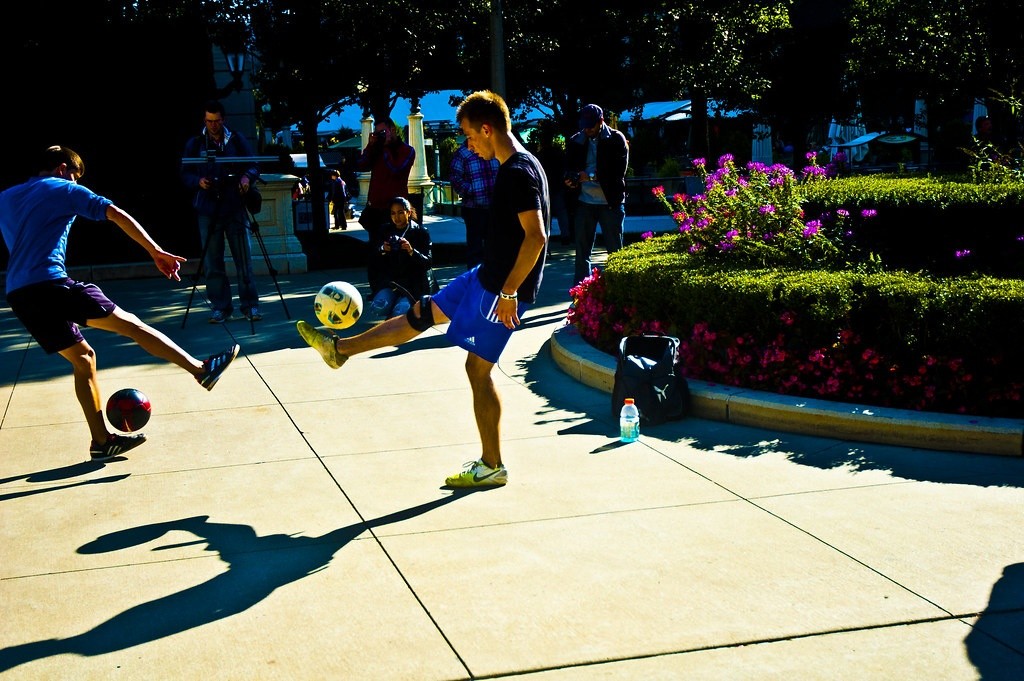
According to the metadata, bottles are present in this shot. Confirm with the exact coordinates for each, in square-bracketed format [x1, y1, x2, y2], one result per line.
[619, 397, 640, 443]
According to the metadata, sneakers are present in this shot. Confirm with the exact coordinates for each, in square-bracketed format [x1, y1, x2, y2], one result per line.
[445, 458, 508, 487]
[195, 343, 241, 391]
[243, 307, 262, 321]
[297, 321, 349, 369]
[89, 433, 146, 460]
[209, 311, 233, 322]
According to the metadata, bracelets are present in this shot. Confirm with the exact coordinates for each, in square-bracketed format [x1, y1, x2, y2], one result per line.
[589, 173, 594, 180]
[500, 290, 517, 300]
[409, 250, 413, 254]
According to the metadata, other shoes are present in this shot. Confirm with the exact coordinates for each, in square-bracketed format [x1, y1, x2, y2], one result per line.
[341, 228, 346, 230]
[331, 227, 339, 230]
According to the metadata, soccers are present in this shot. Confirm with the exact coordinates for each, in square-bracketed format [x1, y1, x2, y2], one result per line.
[107, 388, 151, 433]
[314, 281, 363, 329]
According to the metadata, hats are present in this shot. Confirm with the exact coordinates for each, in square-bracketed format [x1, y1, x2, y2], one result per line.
[577, 104, 602, 128]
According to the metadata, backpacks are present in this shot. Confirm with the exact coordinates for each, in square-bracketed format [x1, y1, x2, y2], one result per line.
[612, 334, 692, 424]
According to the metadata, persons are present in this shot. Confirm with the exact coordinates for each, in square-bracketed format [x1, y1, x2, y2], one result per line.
[0, 145, 241, 463]
[565, 104, 629, 286]
[968, 116, 1002, 153]
[358, 116, 415, 301]
[296, 90, 552, 488]
[301, 171, 310, 186]
[371, 197, 432, 318]
[330, 170, 347, 230]
[180, 102, 265, 323]
[449, 139, 500, 269]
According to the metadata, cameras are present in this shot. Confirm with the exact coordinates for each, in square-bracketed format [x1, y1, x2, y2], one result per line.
[374, 129, 387, 144]
[388, 235, 403, 251]
[564, 171, 579, 186]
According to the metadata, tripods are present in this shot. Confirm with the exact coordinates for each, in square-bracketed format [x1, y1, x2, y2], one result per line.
[182, 175, 292, 335]
